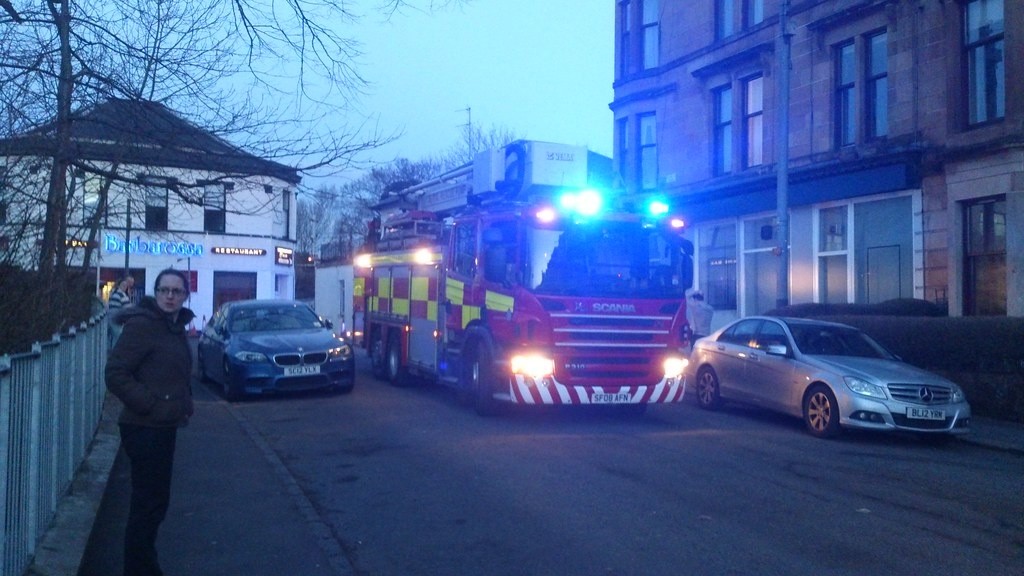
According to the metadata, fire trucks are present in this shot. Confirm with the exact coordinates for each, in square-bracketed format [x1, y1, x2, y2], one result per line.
[351, 139, 695, 420]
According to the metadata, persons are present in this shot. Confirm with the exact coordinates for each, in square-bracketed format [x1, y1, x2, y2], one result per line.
[104, 268, 196, 576]
[685, 287, 714, 351]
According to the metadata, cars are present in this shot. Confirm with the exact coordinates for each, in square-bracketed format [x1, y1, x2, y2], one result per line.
[685, 315, 973, 440]
[198, 298, 356, 403]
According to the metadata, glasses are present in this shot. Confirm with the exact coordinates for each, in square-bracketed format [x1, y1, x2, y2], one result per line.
[155, 285, 187, 296]
